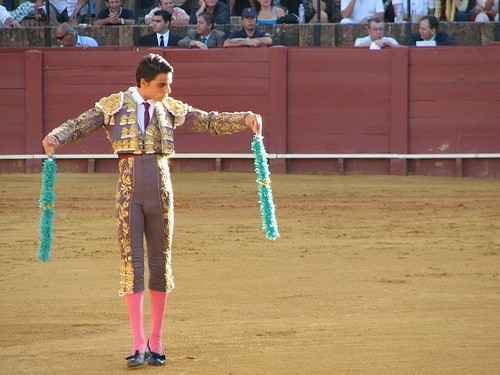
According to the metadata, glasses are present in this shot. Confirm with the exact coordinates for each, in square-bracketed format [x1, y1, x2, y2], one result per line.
[56, 32, 68, 40]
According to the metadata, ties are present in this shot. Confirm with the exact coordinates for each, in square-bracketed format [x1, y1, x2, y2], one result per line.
[160, 36, 165, 47]
[201, 38, 207, 44]
[143, 102, 150, 129]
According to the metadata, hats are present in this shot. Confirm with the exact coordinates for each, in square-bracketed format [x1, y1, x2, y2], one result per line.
[241, 8, 258, 18]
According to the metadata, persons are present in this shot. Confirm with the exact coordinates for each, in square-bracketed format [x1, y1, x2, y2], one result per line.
[0, 0, 500, 50]
[41, 52, 263, 367]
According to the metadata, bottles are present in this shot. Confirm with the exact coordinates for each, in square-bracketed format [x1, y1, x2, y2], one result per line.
[298, 3, 306, 24]
[412, 2, 420, 23]
[397, 1, 403, 23]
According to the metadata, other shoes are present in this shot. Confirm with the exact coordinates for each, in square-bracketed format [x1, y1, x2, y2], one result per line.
[125, 346, 152, 368]
[147, 340, 166, 366]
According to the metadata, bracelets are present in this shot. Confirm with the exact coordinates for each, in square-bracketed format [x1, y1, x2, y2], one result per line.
[77, 5, 81, 9]
[246, 38, 249, 41]
[120, 18, 124, 24]
